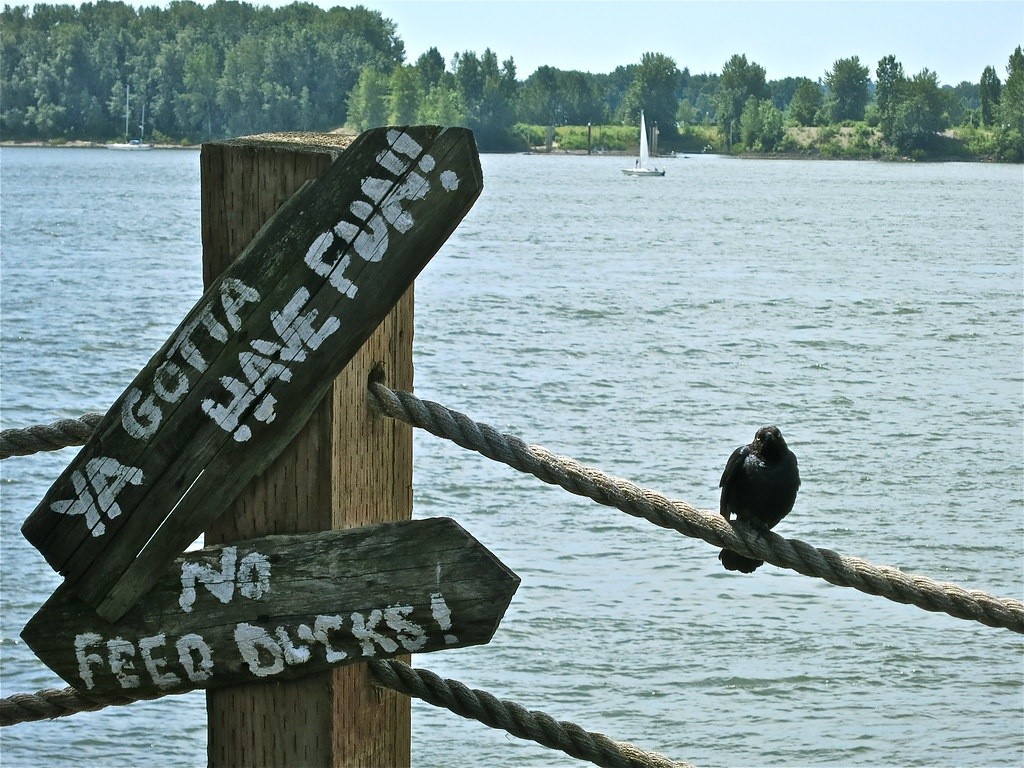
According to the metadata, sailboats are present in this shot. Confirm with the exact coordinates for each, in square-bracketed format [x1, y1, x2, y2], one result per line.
[620, 110, 667, 177]
[106, 83, 152, 149]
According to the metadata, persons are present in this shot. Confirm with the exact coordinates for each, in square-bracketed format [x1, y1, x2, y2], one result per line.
[636, 158, 638, 168]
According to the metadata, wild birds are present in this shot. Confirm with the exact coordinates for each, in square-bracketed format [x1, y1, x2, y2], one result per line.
[718, 426, 801, 574]
